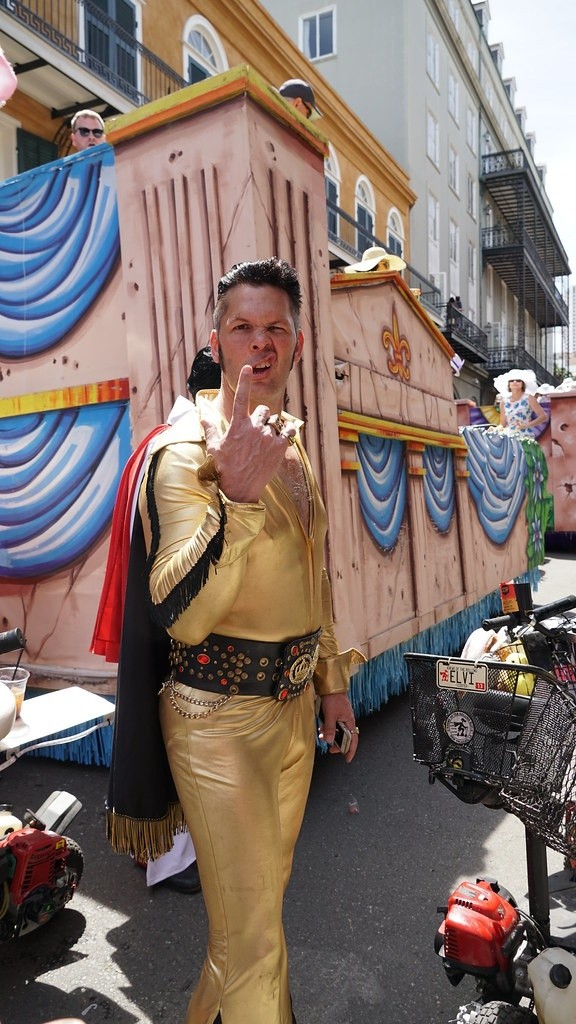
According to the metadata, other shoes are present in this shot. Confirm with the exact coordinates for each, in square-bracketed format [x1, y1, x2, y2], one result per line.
[157, 869, 200, 893]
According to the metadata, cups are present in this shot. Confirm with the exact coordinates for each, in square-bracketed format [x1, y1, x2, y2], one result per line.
[0, 667, 30, 717]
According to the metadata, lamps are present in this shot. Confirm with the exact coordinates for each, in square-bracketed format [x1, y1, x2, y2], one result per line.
[484, 322, 493, 333]
[482, 130, 492, 142]
[483, 204, 493, 215]
[494, 220, 504, 233]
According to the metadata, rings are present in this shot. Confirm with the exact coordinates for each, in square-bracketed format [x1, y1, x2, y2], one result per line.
[282, 432, 296, 445]
[350, 727, 359, 734]
[268, 414, 283, 434]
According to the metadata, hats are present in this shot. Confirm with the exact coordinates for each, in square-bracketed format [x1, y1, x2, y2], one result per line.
[536, 377, 576, 395]
[279, 79, 324, 118]
[493, 369, 538, 396]
[450, 353, 465, 377]
[187, 347, 221, 389]
[344, 247, 407, 273]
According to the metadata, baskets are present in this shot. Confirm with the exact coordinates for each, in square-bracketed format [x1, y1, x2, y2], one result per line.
[499, 675, 576, 861]
[403, 652, 576, 798]
[515, 619, 576, 682]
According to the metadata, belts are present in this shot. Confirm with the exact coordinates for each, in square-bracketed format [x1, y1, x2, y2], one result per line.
[168, 625, 322, 701]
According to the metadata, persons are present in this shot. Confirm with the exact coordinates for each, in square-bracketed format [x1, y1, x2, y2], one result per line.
[345, 246, 407, 274]
[494, 369, 576, 435]
[279, 79, 324, 121]
[92, 257, 358, 1024]
[70, 109, 106, 151]
[447, 296, 463, 329]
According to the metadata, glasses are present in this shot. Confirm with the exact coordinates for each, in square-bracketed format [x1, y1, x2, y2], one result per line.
[509, 380, 522, 382]
[302, 101, 312, 118]
[74, 127, 105, 139]
